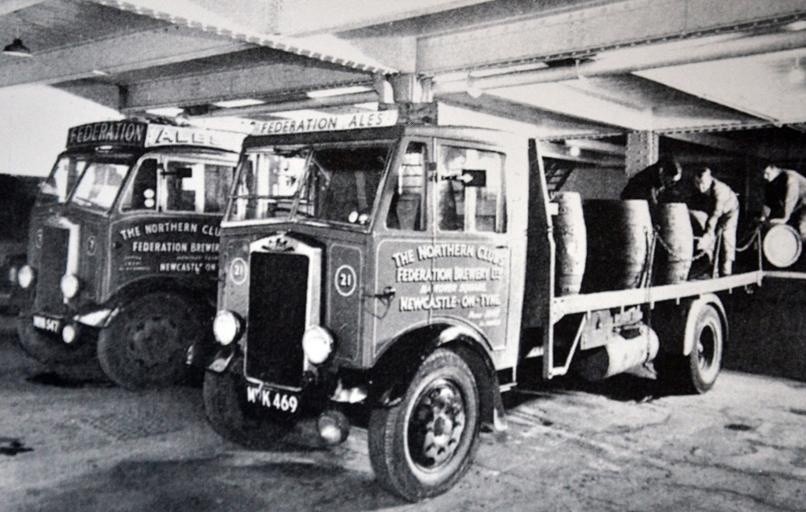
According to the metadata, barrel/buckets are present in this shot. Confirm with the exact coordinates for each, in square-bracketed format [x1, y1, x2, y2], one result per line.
[655, 200, 694, 283]
[552, 191, 587, 295]
[763, 222, 801, 267]
[601, 198, 653, 289]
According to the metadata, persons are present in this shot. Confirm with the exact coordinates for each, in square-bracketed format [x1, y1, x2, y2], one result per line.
[619, 159, 683, 231]
[757, 159, 806, 241]
[689, 167, 740, 275]
